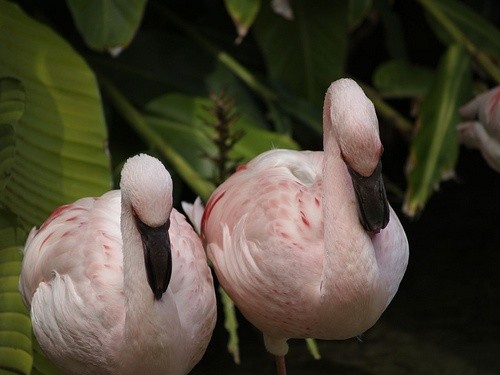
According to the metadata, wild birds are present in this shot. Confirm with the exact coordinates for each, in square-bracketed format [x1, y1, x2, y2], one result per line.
[180, 78, 413, 375]
[452, 85, 500, 173]
[16, 150, 217, 375]
